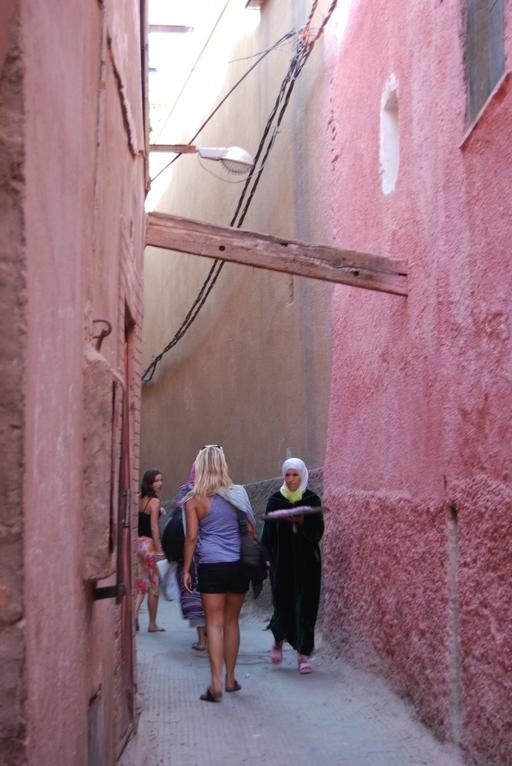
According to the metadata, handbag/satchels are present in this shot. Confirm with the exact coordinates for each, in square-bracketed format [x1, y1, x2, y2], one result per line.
[160, 507, 186, 564]
[236, 535, 268, 584]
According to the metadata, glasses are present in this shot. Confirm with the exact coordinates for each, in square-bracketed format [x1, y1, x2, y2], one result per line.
[200, 444, 221, 451]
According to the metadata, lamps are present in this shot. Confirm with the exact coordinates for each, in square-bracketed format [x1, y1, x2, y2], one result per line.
[193, 147, 256, 174]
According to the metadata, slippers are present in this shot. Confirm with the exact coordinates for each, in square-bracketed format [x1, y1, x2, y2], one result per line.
[135, 618, 312, 703]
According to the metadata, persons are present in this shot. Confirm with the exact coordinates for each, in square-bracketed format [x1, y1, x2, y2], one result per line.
[259, 458, 325, 676]
[166, 457, 209, 651]
[178, 443, 257, 703]
[133, 470, 168, 633]
